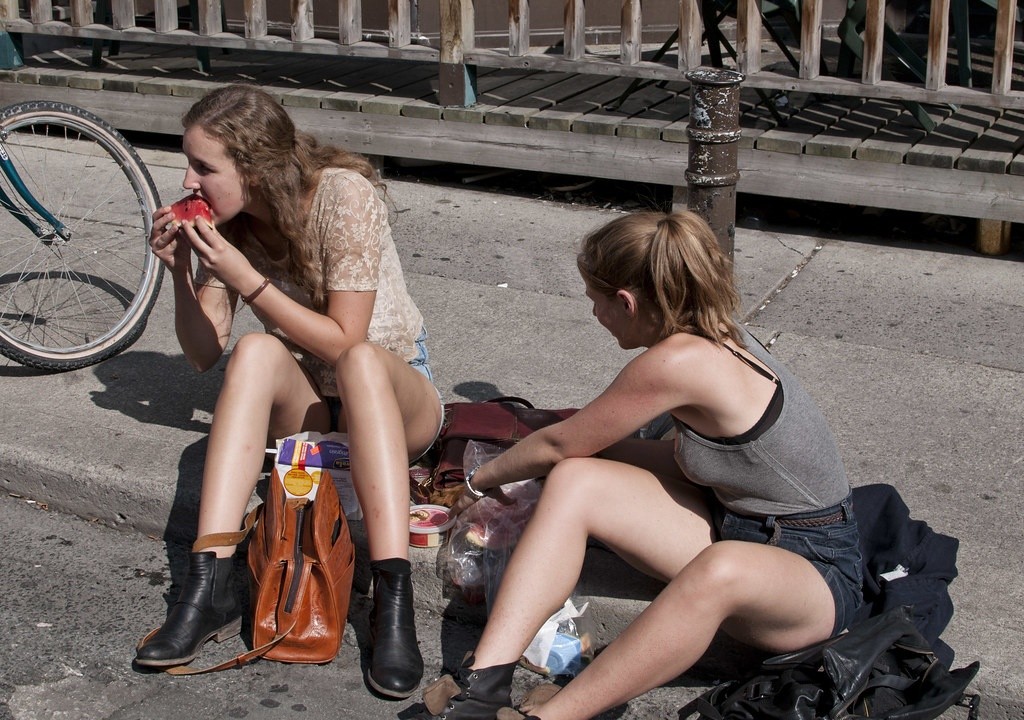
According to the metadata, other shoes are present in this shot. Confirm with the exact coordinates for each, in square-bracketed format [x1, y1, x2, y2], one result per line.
[397, 650, 541, 720]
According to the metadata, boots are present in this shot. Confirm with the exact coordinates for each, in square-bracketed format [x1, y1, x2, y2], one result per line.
[370, 567, 424, 697]
[135, 552, 242, 666]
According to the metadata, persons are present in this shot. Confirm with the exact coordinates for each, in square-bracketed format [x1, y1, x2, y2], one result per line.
[415, 211, 863, 720]
[134, 85, 445, 698]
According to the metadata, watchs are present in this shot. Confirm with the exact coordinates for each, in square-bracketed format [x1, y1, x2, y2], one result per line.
[466, 466, 491, 499]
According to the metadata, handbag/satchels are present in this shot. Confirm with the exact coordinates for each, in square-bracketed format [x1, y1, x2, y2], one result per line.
[135, 466, 354, 674]
[668, 603, 980, 720]
[432, 397, 583, 491]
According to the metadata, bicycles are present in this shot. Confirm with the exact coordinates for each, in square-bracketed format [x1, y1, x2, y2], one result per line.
[0, 101, 165, 373]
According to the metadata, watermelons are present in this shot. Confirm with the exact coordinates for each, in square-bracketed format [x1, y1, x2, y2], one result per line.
[162, 194, 214, 233]
[456, 481, 541, 550]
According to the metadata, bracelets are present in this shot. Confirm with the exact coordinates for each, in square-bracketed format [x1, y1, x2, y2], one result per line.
[241, 274, 271, 303]
